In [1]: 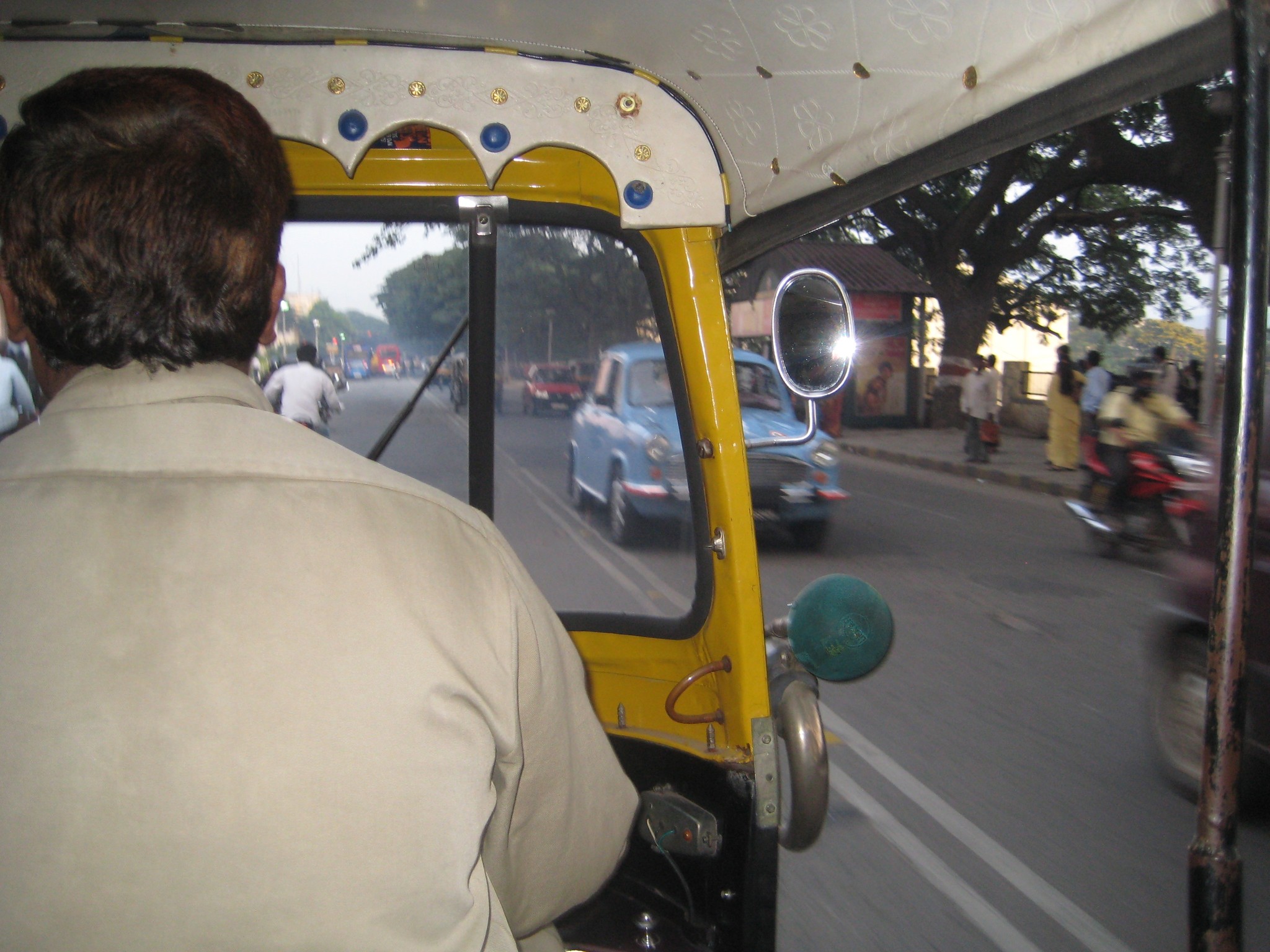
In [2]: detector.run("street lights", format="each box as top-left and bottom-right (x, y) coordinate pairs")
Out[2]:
(280, 301), (288, 365)
(313, 318), (322, 355)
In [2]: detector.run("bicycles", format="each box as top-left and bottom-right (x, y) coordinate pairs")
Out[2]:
(299, 404), (345, 441)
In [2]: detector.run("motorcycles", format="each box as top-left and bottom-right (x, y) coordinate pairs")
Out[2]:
(1063, 419), (1217, 564)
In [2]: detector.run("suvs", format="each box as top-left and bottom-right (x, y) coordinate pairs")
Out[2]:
(571, 339), (847, 553)
(521, 362), (582, 416)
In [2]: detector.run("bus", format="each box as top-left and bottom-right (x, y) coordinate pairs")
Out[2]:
(377, 342), (402, 377)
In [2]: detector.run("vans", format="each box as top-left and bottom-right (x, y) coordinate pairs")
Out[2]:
(451, 351), (502, 415)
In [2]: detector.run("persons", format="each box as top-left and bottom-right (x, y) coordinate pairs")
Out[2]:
(1098, 370), (1203, 536)
(1148, 345), (1180, 403)
(1077, 348), (1112, 435)
(957, 352), (998, 464)
(1055, 345), (1087, 376)
(986, 351), (1001, 424)
(1127, 355), (1211, 422)
(261, 342), (345, 437)
(1044, 362), (1087, 473)
(0, 65), (638, 951)
(0, 337), (40, 440)
(878, 360), (907, 417)
(859, 374), (884, 418)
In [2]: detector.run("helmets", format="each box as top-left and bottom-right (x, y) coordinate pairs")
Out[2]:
(1129, 358), (1164, 394)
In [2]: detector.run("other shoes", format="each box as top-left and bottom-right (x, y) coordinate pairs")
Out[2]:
(967, 448), (997, 464)
(1044, 460), (1063, 471)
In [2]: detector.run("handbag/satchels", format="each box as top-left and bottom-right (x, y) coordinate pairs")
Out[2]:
(980, 422), (999, 445)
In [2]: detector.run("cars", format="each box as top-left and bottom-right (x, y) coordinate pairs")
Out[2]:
(405, 355), (451, 386)
(345, 358), (371, 379)
(1140, 501), (1270, 818)
(323, 364), (347, 389)
